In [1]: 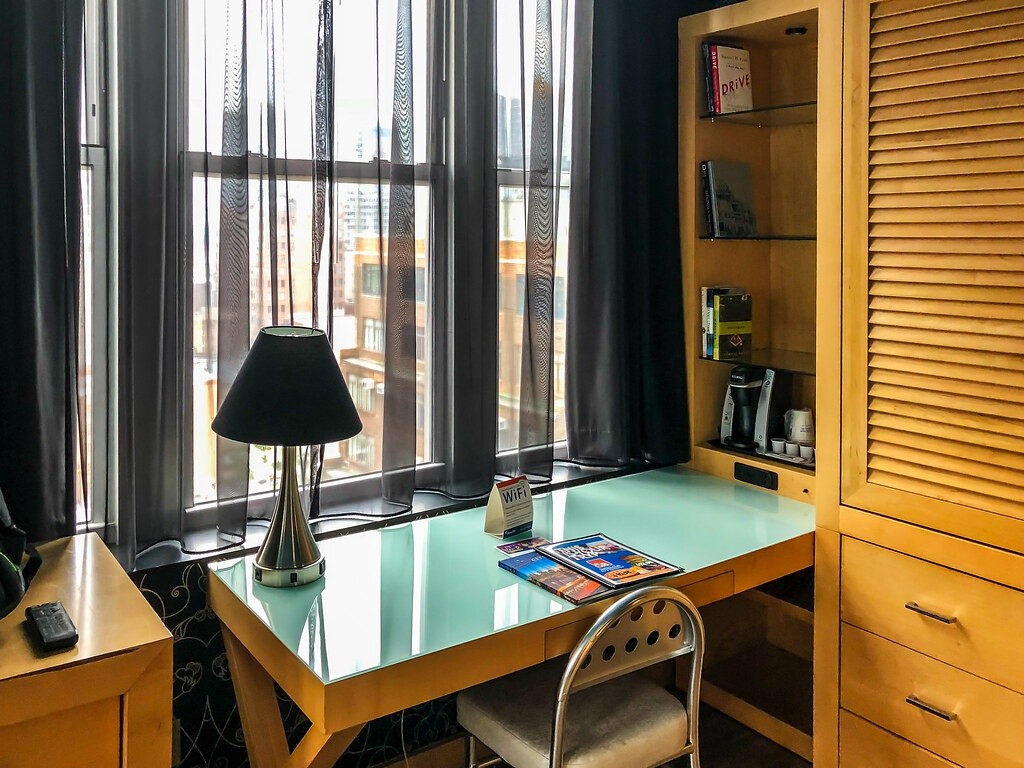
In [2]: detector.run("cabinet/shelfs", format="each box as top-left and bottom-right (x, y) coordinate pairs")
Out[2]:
(679, 0), (1024, 768)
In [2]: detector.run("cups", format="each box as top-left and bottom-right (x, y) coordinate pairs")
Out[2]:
(799, 445), (814, 462)
(784, 441), (799, 457)
(771, 438), (786, 453)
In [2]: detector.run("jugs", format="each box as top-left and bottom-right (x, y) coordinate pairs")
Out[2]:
(788, 409), (814, 446)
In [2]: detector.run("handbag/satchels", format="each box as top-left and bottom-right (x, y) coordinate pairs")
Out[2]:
(0, 492), (42, 619)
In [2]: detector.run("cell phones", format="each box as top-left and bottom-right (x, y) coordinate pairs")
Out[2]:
(24, 600), (79, 650)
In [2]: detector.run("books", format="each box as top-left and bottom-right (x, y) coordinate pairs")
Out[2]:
(701, 160), (758, 238)
(498, 533), (685, 606)
(700, 286), (752, 360)
(701, 40), (754, 120)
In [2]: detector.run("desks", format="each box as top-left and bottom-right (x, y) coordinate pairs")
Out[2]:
(207, 468), (817, 768)
(0, 533), (175, 768)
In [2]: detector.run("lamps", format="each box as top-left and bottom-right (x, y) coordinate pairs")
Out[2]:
(211, 326), (362, 589)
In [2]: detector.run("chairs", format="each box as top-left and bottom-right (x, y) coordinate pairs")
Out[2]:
(458, 586), (706, 768)
